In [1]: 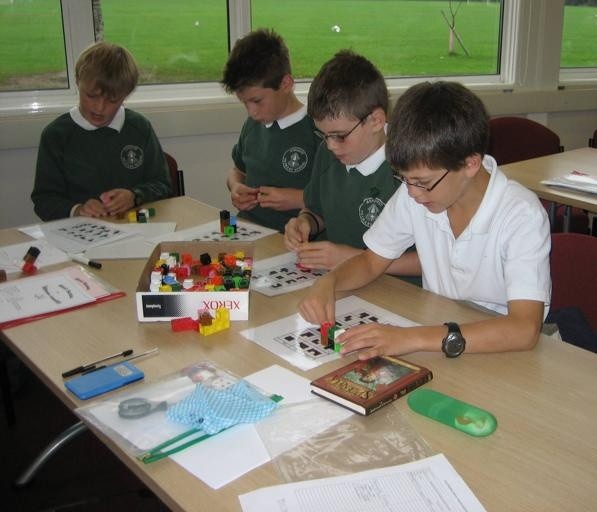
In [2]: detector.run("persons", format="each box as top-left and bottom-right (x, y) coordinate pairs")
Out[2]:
(283, 48), (422, 276)
(219, 27), (326, 233)
(32, 38), (173, 222)
(296, 81), (553, 364)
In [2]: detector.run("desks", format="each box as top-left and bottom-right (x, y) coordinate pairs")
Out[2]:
(496, 146), (597, 235)
(0, 193), (597, 512)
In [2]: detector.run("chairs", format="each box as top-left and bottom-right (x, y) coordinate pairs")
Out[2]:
(156, 151), (185, 198)
(486, 116), (588, 230)
(541, 229), (597, 355)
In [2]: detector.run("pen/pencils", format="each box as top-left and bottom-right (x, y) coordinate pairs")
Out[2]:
(72, 255), (101, 269)
(62, 348), (160, 378)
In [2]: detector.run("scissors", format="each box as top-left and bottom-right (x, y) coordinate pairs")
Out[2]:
(119, 398), (167, 419)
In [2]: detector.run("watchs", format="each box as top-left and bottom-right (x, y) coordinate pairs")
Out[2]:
(441, 321), (465, 359)
(131, 188), (144, 207)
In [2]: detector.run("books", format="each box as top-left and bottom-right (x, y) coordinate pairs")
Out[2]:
(309, 352), (433, 418)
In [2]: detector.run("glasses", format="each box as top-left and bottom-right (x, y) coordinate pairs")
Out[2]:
(313, 111), (372, 143)
(392, 167), (450, 195)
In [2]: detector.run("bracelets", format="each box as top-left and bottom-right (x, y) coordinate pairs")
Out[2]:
(298, 211), (321, 235)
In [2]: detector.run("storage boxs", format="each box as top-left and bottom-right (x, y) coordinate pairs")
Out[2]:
(134, 238), (254, 323)
